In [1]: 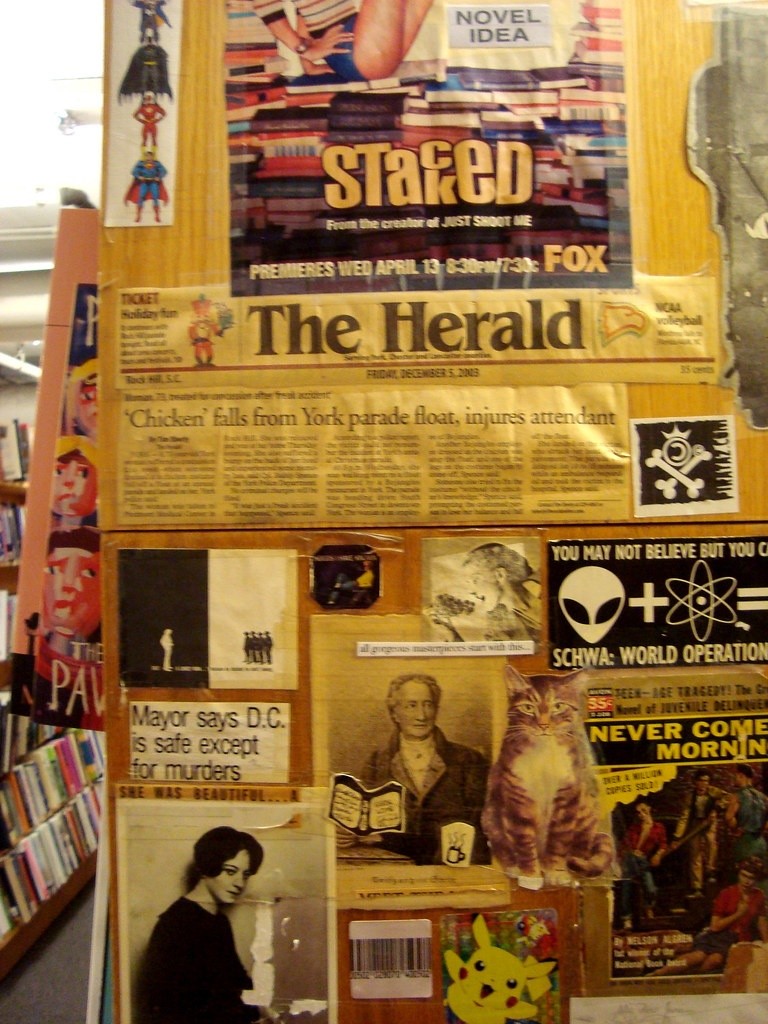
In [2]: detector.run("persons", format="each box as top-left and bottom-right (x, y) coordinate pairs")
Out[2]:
(669, 768), (731, 900)
(356, 673), (491, 867)
(428, 543), (542, 643)
(617, 800), (667, 933)
(724, 766), (766, 880)
(137, 828), (283, 1024)
(645, 853), (767, 978)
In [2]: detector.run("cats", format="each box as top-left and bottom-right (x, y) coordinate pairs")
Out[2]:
(480, 664), (617, 889)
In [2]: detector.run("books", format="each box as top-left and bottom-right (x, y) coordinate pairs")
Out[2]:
(226, 1), (633, 278)
(0, 416), (105, 937)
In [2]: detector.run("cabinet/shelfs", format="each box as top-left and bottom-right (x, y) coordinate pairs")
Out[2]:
(1, 351), (107, 991)
